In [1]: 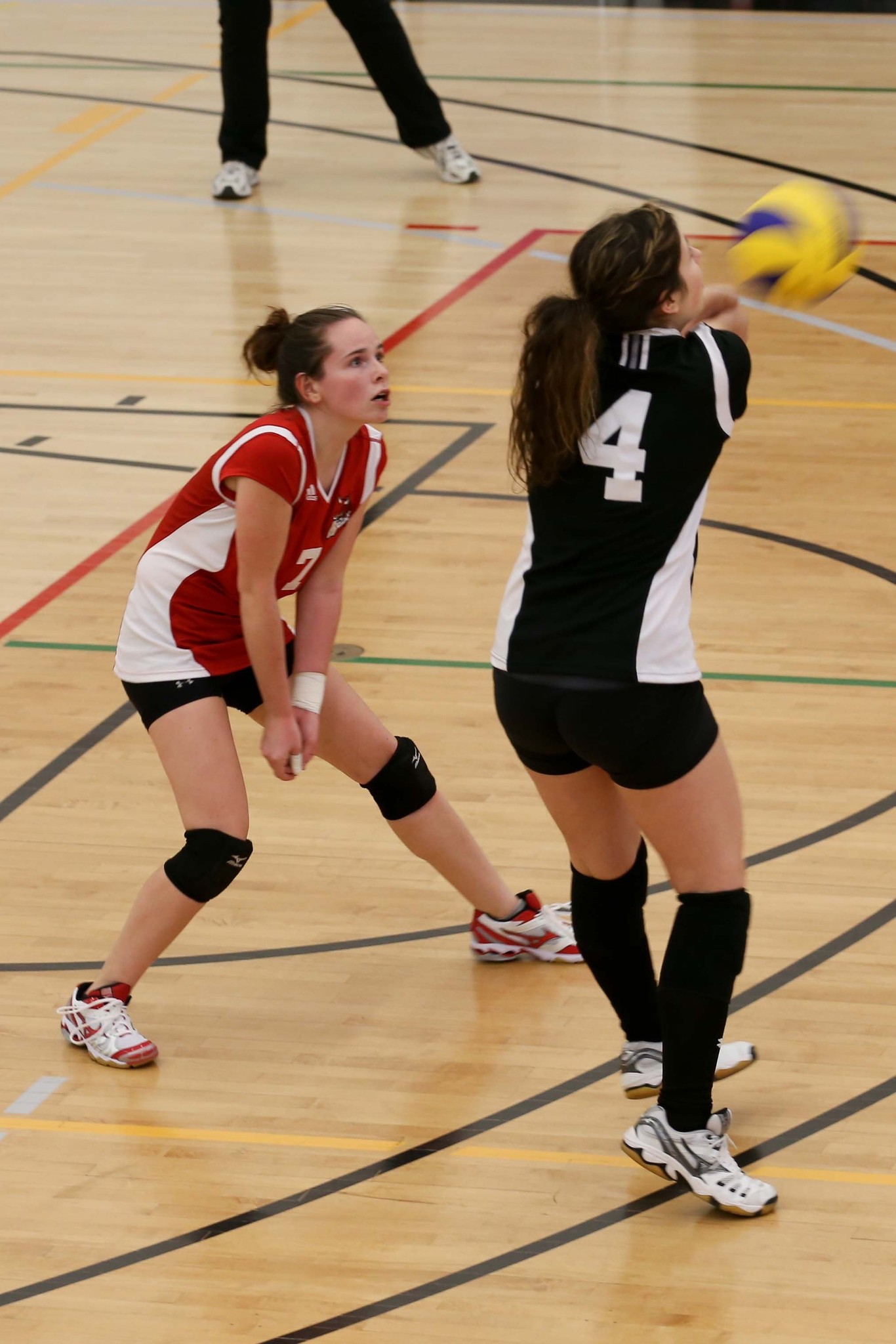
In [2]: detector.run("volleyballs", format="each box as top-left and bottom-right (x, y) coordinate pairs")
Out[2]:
(728, 180), (865, 308)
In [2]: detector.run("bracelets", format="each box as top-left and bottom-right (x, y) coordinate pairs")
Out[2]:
(289, 672), (326, 715)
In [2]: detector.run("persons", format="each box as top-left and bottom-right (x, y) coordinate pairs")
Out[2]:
(55, 303), (584, 1069)
(212, 0), (480, 199)
(489, 200), (780, 1216)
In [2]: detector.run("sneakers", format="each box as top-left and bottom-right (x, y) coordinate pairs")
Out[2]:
(619, 1107), (778, 1216)
(212, 160), (259, 198)
(413, 133), (482, 184)
(620, 1039), (757, 1098)
(57, 981), (158, 1068)
(471, 888), (586, 962)
